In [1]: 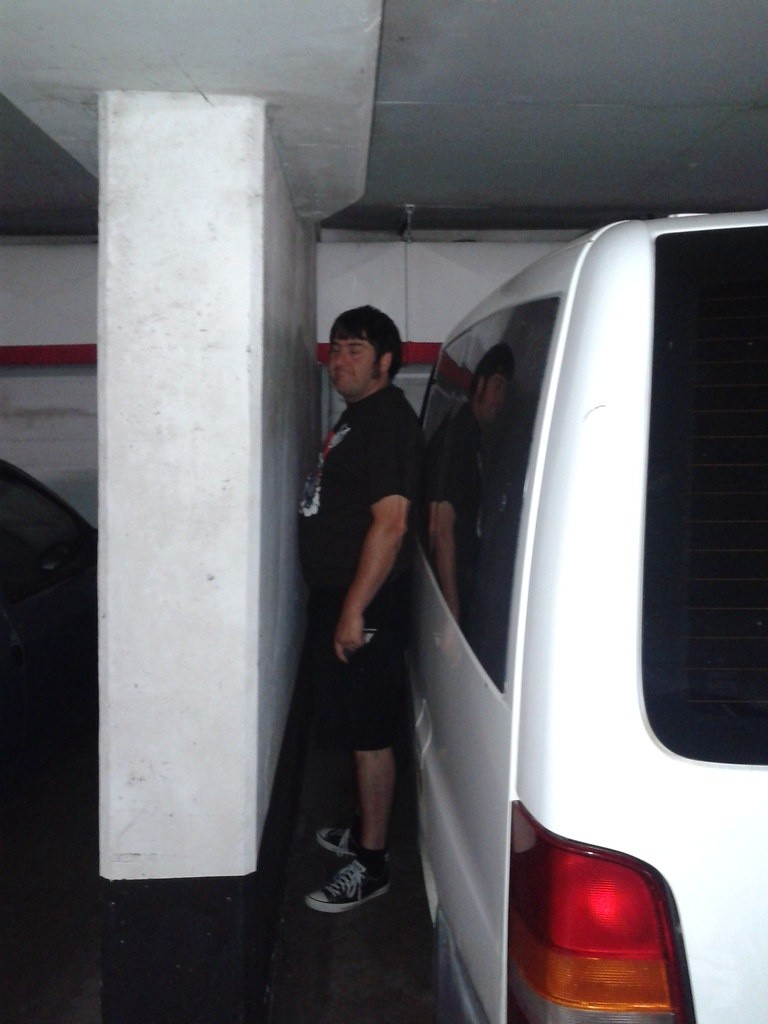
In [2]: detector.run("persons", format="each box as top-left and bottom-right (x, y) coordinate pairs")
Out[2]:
(299, 304), (422, 911)
(423, 341), (514, 626)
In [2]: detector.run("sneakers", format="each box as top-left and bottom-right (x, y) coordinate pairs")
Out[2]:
(304, 860), (391, 913)
(316, 827), (390, 863)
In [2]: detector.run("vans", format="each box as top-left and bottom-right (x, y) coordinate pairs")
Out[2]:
(391, 210), (768, 1024)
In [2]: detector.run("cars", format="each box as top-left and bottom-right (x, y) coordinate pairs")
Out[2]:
(0, 458), (101, 911)
